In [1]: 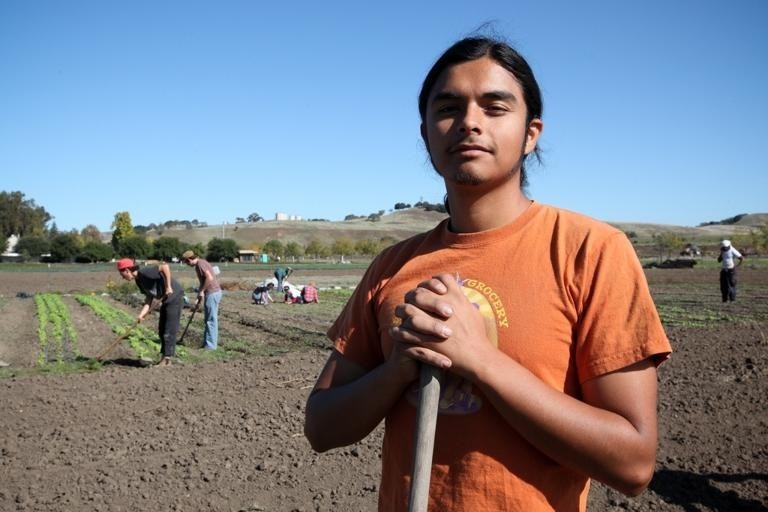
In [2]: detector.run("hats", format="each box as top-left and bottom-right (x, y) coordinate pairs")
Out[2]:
(181, 250), (194, 263)
(117, 257), (133, 270)
(722, 240), (729, 247)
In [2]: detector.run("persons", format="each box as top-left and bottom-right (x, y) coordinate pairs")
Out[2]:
(302, 20), (675, 512)
(300, 280), (320, 304)
(274, 266), (293, 292)
(181, 250), (223, 351)
(282, 285), (302, 304)
(717, 239), (745, 303)
(252, 282), (277, 306)
(117, 258), (185, 367)
